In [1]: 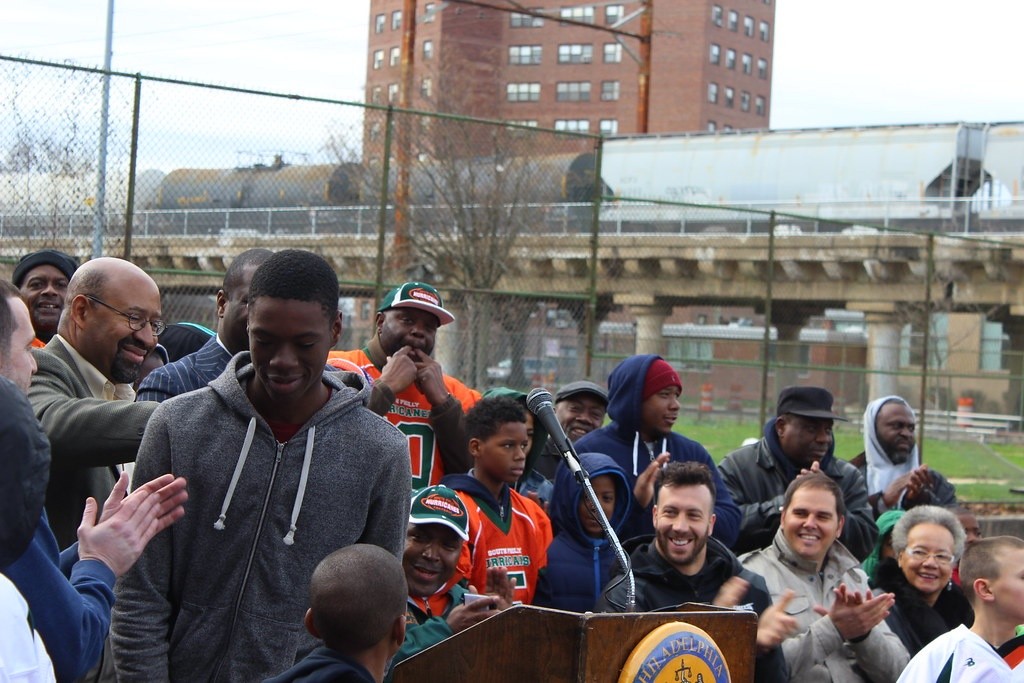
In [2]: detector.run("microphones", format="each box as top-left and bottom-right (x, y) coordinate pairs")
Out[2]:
(527, 388), (584, 485)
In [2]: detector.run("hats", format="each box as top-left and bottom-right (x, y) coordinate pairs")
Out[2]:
(11, 249), (80, 292)
(777, 386), (848, 422)
(555, 380), (609, 410)
(408, 482), (471, 543)
(377, 281), (454, 325)
(642, 357), (683, 404)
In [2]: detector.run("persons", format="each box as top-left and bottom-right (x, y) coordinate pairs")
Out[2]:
(12, 248), (274, 683)
(718, 385), (1024, 683)
(263, 544), (409, 683)
(0, 375), (56, 683)
(444, 354), (798, 683)
(109, 250), (412, 683)
(0, 278), (188, 683)
(383, 484), (517, 683)
(326, 281), (478, 492)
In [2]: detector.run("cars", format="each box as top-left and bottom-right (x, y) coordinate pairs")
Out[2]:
(486, 354), (560, 389)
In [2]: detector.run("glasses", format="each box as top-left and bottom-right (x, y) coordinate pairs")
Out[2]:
(83, 295), (168, 336)
(903, 544), (956, 565)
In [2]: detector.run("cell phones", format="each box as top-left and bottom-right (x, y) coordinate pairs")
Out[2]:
(463, 593), (490, 611)
